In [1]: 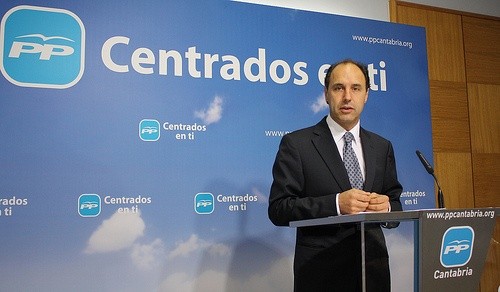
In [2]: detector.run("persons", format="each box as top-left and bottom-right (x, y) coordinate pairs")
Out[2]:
(267, 58), (403, 292)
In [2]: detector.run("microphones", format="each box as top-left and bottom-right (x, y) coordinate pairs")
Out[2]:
(415, 150), (444, 209)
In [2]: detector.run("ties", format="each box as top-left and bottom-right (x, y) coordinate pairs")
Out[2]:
(342, 131), (363, 190)
